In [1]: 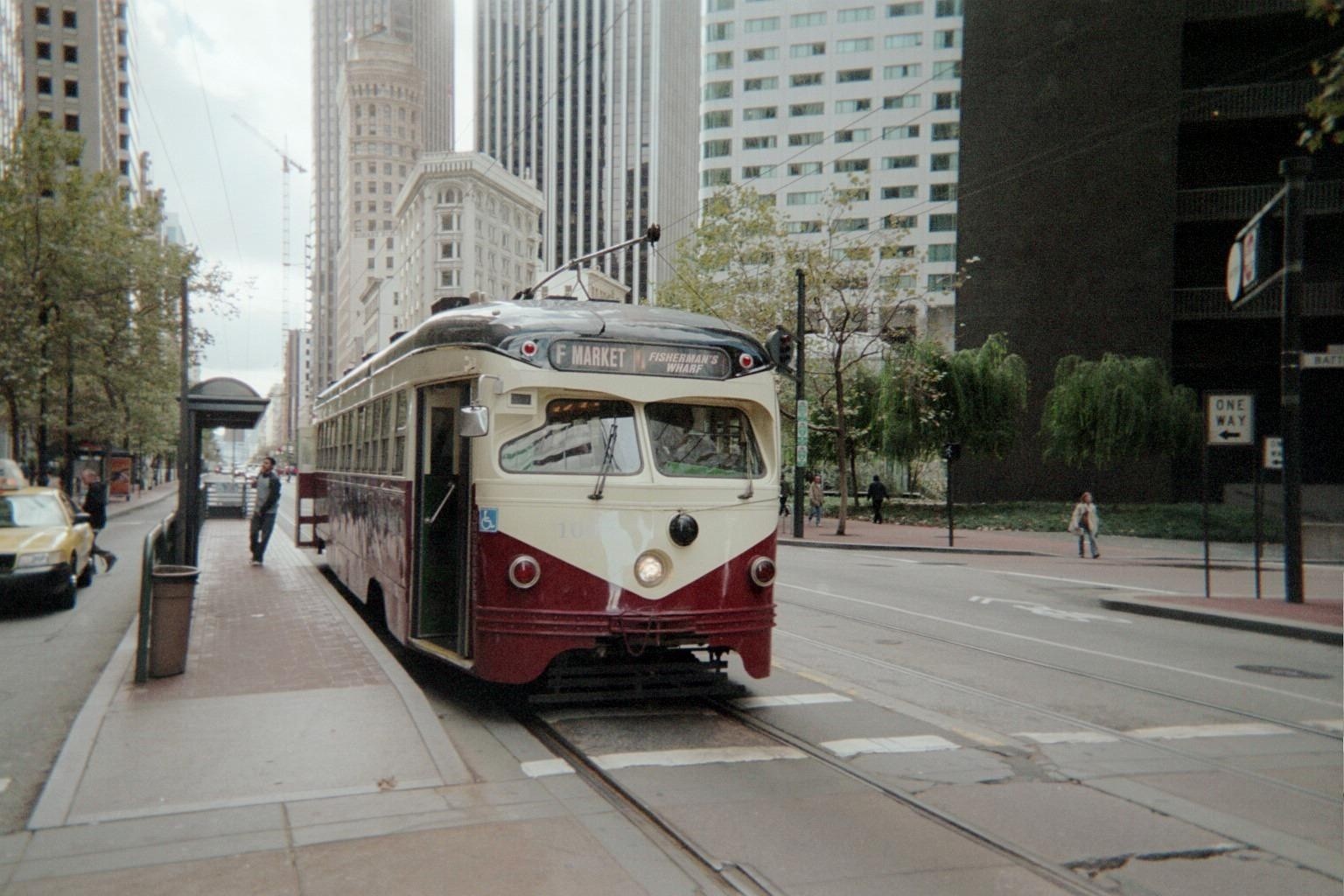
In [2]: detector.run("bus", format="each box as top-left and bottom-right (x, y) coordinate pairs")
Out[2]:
(308, 291), (785, 713)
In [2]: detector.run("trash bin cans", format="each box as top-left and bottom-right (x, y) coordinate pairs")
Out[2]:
(148, 565), (200, 678)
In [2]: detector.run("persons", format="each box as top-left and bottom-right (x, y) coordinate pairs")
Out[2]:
(808, 474), (824, 528)
(867, 474), (890, 523)
(249, 455), (283, 564)
(653, 405), (723, 468)
(1067, 491), (1101, 560)
(79, 467), (121, 574)
(779, 472), (792, 516)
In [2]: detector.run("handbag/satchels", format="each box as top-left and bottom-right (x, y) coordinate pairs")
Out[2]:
(1071, 526), (1084, 536)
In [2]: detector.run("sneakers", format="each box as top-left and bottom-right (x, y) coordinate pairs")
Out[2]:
(1092, 553), (1100, 559)
(104, 552), (117, 573)
(1078, 556), (1085, 558)
(250, 558), (264, 567)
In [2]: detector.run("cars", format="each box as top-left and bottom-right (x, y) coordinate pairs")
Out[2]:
(2, 458), (93, 613)
